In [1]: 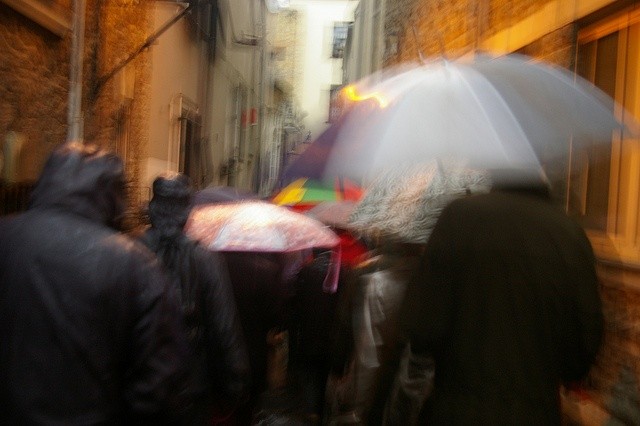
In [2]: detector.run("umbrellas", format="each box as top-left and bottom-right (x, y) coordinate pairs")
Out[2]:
(278, 176), (364, 208)
(341, 162), (504, 244)
(323, 53), (635, 190)
(191, 188), (261, 204)
(187, 199), (340, 252)
(276, 113), (344, 185)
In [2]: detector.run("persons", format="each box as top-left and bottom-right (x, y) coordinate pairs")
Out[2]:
(137, 176), (252, 424)
(291, 230), (354, 423)
(388, 173), (604, 424)
(340, 237), (424, 425)
(1, 141), (185, 426)
(225, 252), (290, 425)
